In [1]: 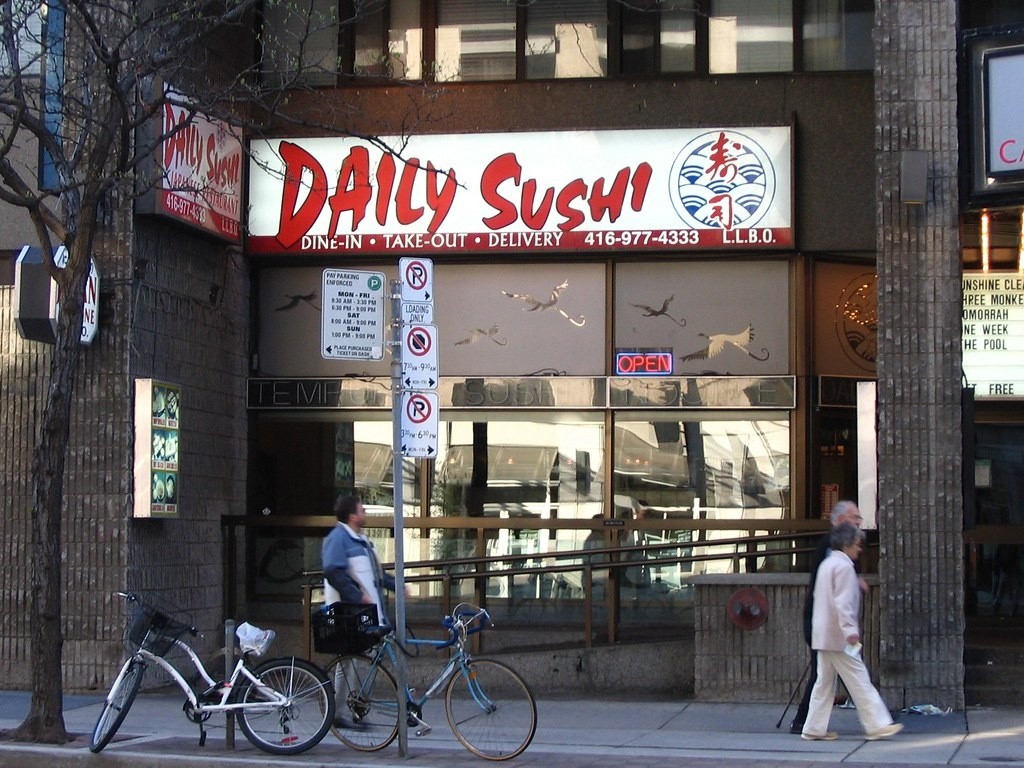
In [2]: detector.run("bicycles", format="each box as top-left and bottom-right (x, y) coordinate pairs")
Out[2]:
(89, 590), (337, 756)
(317, 600), (538, 761)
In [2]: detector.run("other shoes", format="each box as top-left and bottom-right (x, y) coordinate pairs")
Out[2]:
(865, 723), (904, 741)
(802, 731), (838, 740)
(790, 721), (803, 733)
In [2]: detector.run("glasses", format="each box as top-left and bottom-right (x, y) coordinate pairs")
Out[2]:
(853, 541), (864, 549)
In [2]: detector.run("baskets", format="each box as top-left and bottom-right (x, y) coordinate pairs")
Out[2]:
(129, 595), (194, 657)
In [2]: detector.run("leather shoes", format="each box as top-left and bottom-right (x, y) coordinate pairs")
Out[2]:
(333, 716), (369, 730)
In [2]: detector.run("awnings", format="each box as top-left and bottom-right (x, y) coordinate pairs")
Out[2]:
(354, 442), (395, 489)
(439, 444), (560, 488)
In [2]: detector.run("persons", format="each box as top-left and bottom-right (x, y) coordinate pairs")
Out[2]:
(582, 516), (608, 597)
(322, 497), (413, 729)
(791, 503), (903, 741)
